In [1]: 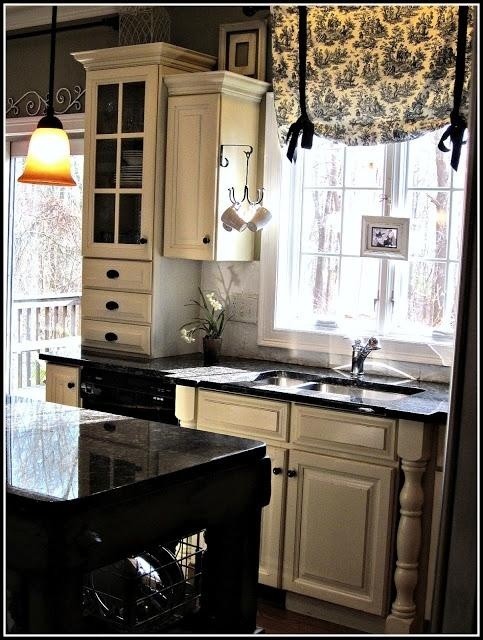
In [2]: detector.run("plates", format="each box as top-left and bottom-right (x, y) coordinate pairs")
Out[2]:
(87, 545), (188, 628)
(111, 148), (143, 188)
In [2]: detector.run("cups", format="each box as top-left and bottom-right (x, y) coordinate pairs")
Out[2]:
(220, 206), (247, 233)
(247, 207), (272, 233)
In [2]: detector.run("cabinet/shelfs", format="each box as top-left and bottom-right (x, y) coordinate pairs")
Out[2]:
(162, 69), (267, 262)
(69, 45), (200, 357)
(39, 360), (443, 635)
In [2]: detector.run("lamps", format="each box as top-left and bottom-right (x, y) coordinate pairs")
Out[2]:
(16, 1), (74, 187)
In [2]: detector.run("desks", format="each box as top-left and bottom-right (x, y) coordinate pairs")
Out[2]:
(6, 394), (266, 636)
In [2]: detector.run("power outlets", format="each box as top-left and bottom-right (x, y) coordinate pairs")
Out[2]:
(228, 295), (257, 324)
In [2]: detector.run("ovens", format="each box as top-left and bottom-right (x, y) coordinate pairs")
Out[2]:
(79, 369), (177, 427)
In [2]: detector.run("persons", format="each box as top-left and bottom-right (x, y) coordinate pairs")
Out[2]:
(387, 230), (395, 245)
(375, 231), (392, 247)
(381, 233), (387, 240)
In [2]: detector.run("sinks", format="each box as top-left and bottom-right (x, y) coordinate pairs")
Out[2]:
(249, 369), (314, 388)
(298, 377), (426, 401)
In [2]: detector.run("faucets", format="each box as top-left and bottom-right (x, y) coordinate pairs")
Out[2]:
(350, 336), (381, 375)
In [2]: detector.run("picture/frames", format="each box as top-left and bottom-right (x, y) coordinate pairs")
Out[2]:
(216, 19), (265, 83)
(360, 213), (409, 260)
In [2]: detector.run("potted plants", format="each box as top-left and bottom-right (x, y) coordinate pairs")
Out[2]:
(181, 287), (233, 359)
